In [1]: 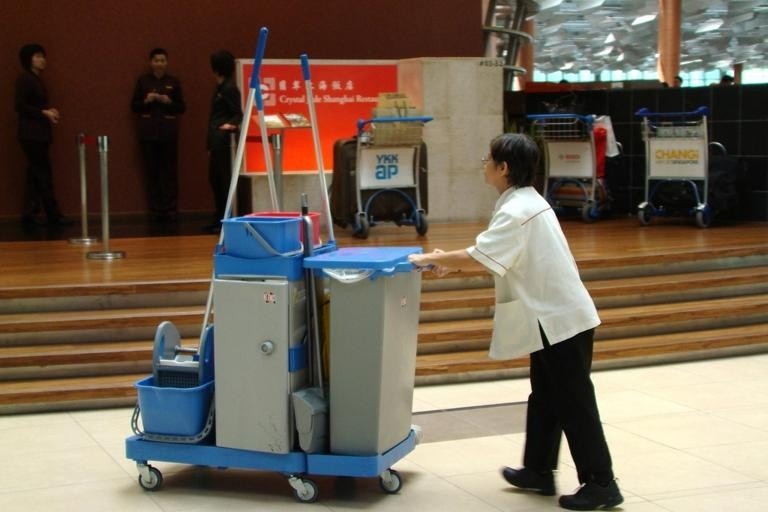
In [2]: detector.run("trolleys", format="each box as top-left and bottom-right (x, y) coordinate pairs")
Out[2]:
(528, 111), (612, 220)
(99, 247), (436, 495)
(345, 113), (431, 234)
(630, 103), (738, 227)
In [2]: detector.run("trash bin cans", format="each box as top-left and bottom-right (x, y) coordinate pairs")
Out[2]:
(303, 246), (423, 457)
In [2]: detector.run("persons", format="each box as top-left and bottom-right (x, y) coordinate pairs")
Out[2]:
(13, 42), (70, 231)
(411, 133), (626, 510)
(131, 46), (181, 221)
(204, 47), (251, 230)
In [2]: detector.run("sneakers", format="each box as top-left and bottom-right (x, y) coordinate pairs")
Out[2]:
(146, 215), (183, 234)
(201, 219), (222, 233)
(21, 211), (78, 233)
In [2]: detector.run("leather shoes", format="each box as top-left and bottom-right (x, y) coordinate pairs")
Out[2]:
(503, 466), (556, 495)
(559, 480), (624, 510)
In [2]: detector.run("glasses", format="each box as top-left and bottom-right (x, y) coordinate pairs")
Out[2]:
(481, 156), (496, 163)
